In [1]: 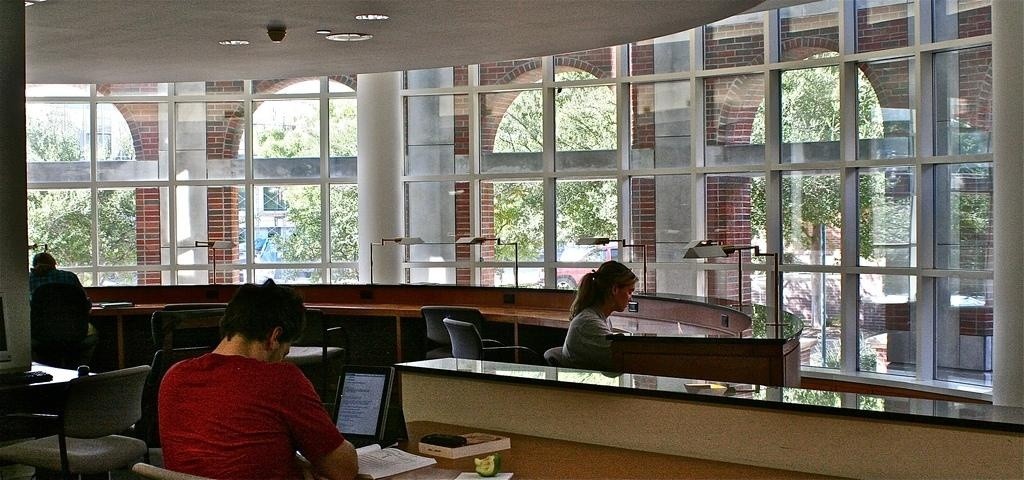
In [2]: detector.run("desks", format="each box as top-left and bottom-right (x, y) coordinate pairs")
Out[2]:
(295, 419), (854, 480)
(81, 300), (740, 408)
(0, 361), (97, 393)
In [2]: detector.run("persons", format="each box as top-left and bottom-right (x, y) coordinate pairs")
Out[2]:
(157, 283), (358, 479)
(29, 252), (90, 363)
(562, 260), (640, 364)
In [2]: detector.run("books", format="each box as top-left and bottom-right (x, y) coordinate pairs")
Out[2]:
(419, 432), (511, 459)
(354, 443), (437, 480)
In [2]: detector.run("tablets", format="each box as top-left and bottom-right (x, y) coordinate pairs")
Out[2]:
(332, 365), (395, 442)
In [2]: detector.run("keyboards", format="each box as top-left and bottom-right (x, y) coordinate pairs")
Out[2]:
(1, 371), (54, 386)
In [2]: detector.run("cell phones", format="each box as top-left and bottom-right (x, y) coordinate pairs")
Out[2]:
(420, 433), (466, 447)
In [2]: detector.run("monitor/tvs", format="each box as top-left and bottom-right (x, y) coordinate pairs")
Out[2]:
(0, 293), (16, 363)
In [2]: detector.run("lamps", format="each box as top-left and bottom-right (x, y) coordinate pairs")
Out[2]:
(680, 239), (779, 308)
(370, 237), (424, 285)
(576, 238), (647, 294)
(455, 237), (518, 288)
(195, 241), (237, 265)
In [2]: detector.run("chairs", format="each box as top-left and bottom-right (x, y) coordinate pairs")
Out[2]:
(132, 462), (217, 480)
(0, 364), (151, 480)
(281, 308), (345, 402)
(443, 318), (541, 365)
(141, 303), (230, 422)
(421, 305), (507, 361)
